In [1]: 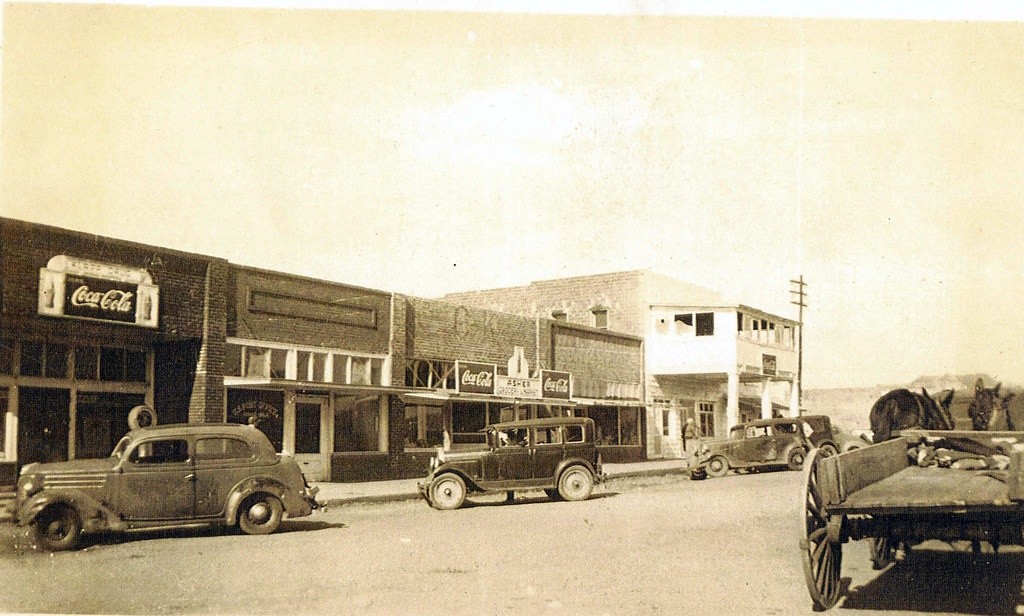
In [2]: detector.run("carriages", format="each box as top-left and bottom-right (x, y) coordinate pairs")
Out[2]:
(798, 378), (1023, 610)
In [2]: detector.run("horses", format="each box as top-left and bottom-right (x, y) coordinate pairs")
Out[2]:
(869, 378), (1016, 445)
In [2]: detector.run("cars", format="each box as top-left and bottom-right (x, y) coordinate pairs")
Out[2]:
(11, 421), (323, 550)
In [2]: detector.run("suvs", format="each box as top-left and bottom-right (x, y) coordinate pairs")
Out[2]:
(416, 416), (609, 510)
(794, 416), (841, 464)
(695, 413), (819, 478)
